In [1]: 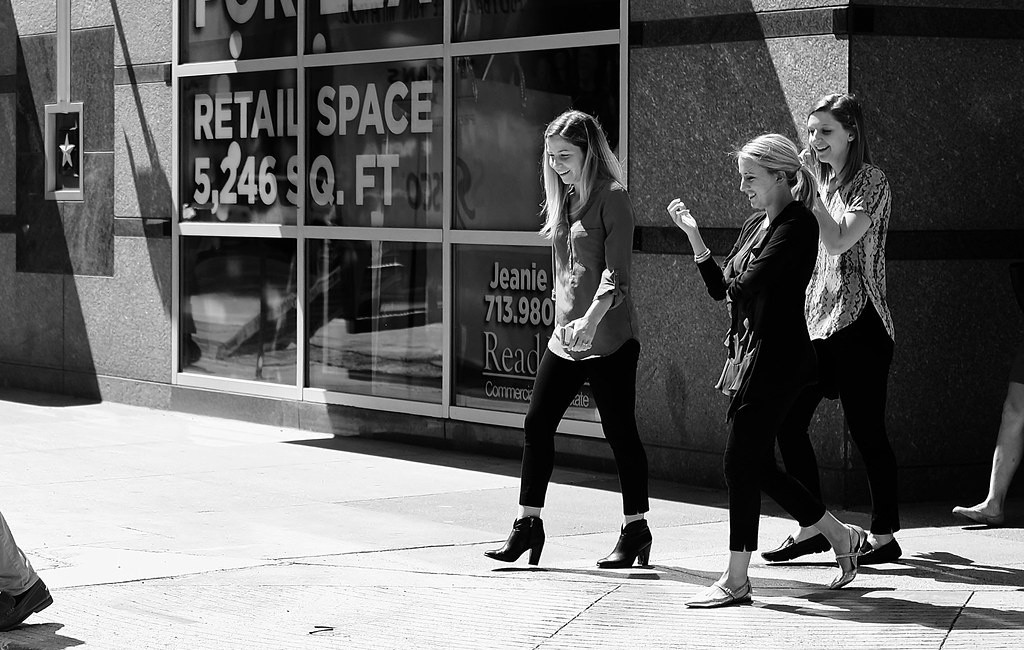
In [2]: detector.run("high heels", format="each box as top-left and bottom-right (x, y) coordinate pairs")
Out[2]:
(484, 516), (545, 565)
(597, 519), (652, 568)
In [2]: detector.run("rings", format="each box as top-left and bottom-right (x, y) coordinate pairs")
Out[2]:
(582, 342), (592, 348)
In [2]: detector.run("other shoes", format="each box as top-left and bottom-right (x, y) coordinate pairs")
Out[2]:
(857, 538), (902, 564)
(953, 506), (1005, 526)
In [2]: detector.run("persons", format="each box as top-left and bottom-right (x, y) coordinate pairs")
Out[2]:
(952, 157), (1024, 527)
(0, 0), (101, 632)
(485, 111), (652, 569)
(761, 95), (902, 567)
(667, 133), (868, 609)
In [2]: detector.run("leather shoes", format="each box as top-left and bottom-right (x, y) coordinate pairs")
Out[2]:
(684, 576), (753, 607)
(830, 523), (868, 589)
(0, 578), (53, 632)
(761, 533), (832, 562)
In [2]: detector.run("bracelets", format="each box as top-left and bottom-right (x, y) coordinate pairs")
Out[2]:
(695, 249), (711, 261)
(816, 191), (820, 197)
